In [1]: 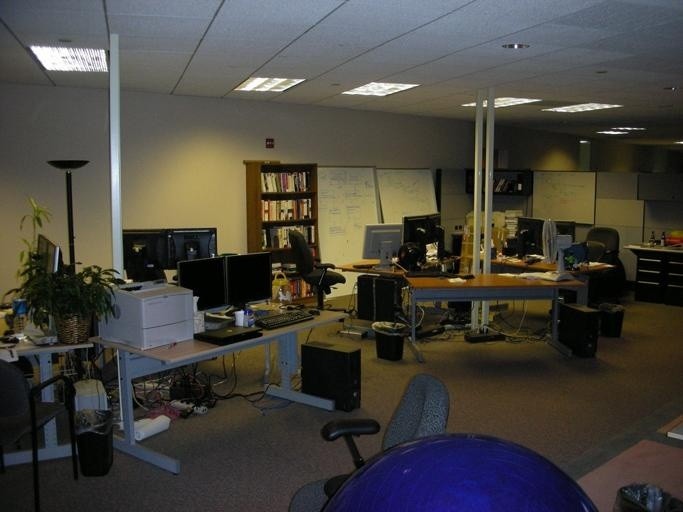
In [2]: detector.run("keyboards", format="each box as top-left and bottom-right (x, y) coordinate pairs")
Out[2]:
(255, 311), (314, 329)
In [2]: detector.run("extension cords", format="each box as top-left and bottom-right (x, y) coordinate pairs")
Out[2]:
(171, 400), (208, 416)
(463, 331), (507, 344)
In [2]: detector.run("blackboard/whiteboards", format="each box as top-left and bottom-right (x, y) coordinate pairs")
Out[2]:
(531, 169), (596, 225)
(377, 169), (437, 223)
(318, 166), (382, 300)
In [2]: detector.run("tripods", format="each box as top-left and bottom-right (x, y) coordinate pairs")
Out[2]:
(177, 256), (226, 319)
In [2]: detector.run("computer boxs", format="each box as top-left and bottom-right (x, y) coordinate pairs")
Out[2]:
(560, 302), (600, 358)
(358, 275), (400, 322)
(301, 341), (361, 410)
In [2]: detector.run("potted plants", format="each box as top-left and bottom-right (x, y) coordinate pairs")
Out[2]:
(20, 266), (126, 345)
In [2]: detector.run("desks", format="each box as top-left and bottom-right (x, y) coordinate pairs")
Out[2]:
(335, 255), (462, 328)
(487, 247), (615, 330)
(405, 274), (584, 363)
(87, 304), (350, 476)
(0, 330), (95, 467)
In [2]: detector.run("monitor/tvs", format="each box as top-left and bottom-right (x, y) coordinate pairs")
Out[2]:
(403, 213), (446, 261)
(167, 228), (217, 281)
(122, 228), (166, 282)
(363, 223), (403, 271)
(513, 217), (575, 263)
(226, 251), (273, 318)
(36, 235), (65, 333)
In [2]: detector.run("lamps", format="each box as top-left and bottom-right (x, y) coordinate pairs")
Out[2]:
(48, 160), (90, 274)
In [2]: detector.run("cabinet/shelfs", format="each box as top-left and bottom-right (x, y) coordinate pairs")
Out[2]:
(625, 249), (683, 307)
(464, 167), (533, 196)
(246, 158), (320, 305)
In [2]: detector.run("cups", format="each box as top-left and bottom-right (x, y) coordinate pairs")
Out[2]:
(12, 298), (26, 314)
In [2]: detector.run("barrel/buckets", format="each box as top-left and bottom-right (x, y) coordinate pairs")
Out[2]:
(272, 271), (289, 300)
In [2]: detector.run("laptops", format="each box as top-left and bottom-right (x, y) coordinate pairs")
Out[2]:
(194, 327), (263, 347)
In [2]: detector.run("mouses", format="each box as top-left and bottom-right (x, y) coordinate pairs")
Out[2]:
(309, 310), (320, 316)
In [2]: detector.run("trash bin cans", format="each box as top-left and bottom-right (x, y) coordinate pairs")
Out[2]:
(75, 410), (114, 477)
(600, 303), (624, 338)
(373, 321), (406, 361)
(616, 485), (683, 512)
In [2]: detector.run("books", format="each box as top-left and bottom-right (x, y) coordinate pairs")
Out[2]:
(260, 171), (315, 300)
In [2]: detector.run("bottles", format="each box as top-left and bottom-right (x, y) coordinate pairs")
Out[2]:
(243, 309), (255, 328)
(649, 231), (655, 247)
(661, 232), (666, 246)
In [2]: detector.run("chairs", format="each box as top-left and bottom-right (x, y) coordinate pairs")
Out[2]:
(287, 373), (451, 507)
(586, 226), (620, 258)
(319, 434), (599, 511)
(286, 229), (346, 314)
(1, 358), (79, 511)
